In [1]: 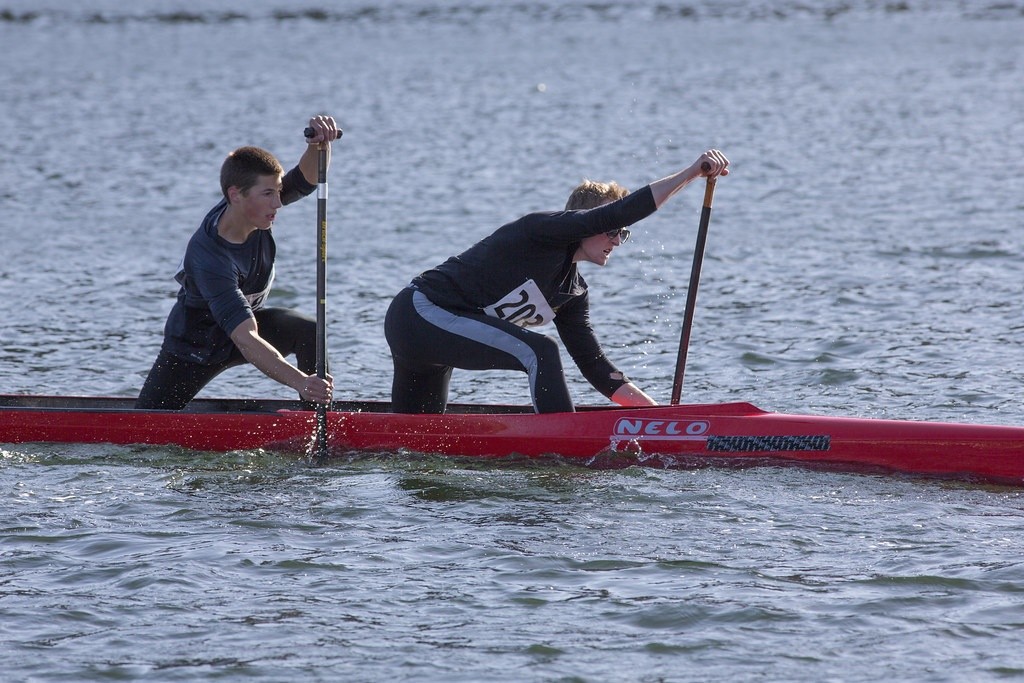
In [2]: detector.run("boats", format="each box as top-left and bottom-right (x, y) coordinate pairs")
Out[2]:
(0, 386), (1024, 481)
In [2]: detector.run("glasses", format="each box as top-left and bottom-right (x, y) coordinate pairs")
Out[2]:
(605, 229), (631, 244)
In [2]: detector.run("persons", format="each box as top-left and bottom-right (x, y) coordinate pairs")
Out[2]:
(134, 114), (338, 411)
(385, 148), (730, 416)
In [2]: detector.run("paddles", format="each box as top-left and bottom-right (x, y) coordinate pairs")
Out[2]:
(302, 124), (348, 468)
(667, 162), (721, 408)
